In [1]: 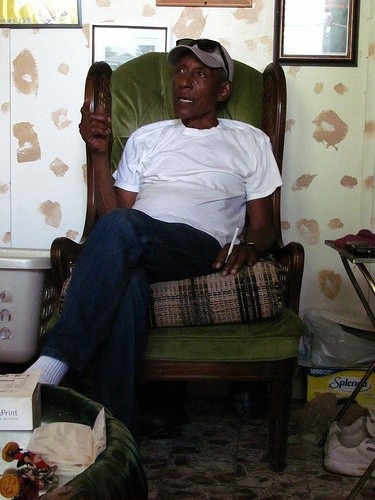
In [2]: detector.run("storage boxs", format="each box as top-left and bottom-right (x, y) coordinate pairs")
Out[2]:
(1, 372), (42, 430)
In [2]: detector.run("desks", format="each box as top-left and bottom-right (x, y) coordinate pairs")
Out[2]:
(0, 374), (148, 500)
(318, 240), (375, 443)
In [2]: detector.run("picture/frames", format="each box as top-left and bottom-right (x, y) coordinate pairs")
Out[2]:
(275, 0), (361, 68)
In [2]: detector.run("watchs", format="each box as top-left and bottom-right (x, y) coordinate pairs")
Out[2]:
(238, 241), (259, 255)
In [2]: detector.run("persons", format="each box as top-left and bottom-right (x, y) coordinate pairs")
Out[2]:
(20, 37), (284, 445)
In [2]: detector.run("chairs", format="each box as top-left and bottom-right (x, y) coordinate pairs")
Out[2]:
(48, 51), (304, 474)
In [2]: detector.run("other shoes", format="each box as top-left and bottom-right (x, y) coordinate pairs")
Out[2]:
(323, 408), (375, 478)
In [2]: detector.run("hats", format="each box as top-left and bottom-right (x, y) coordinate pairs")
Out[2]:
(168, 40), (235, 82)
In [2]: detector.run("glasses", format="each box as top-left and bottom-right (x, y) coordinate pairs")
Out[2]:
(175, 38), (230, 81)
